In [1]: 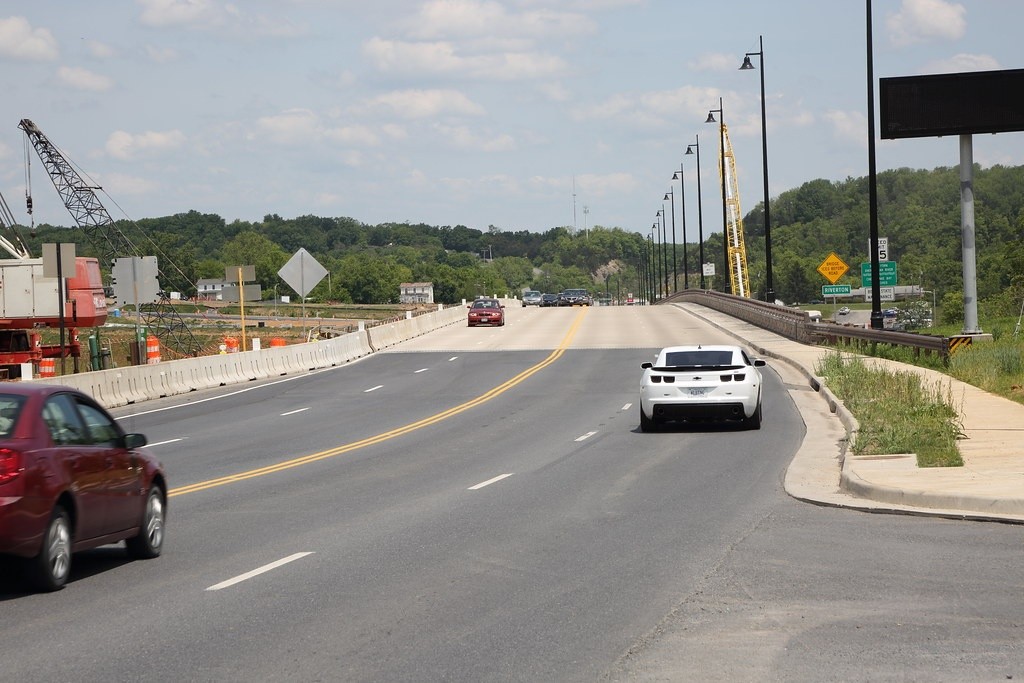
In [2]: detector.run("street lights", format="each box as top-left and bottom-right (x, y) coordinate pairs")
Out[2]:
(655, 204), (668, 298)
(737, 35), (775, 305)
(684, 134), (706, 288)
(663, 186), (677, 293)
(670, 163), (689, 289)
(646, 233), (657, 302)
(704, 97), (733, 294)
(652, 218), (662, 300)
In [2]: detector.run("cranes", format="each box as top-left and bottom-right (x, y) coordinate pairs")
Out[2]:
(16, 116), (222, 362)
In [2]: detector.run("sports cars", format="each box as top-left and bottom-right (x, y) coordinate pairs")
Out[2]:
(639, 345), (766, 429)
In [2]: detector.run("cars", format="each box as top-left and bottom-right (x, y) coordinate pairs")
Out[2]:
(522, 291), (544, 308)
(467, 298), (506, 327)
(840, 307), (850, 315)
(882, 307), (911, 318)
(0, 382), (170, 590)
(541, 294), (558, 307)
(558, 288), (593, 307)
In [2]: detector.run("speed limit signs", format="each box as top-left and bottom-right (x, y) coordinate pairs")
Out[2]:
(868, 238), (890, 263)
